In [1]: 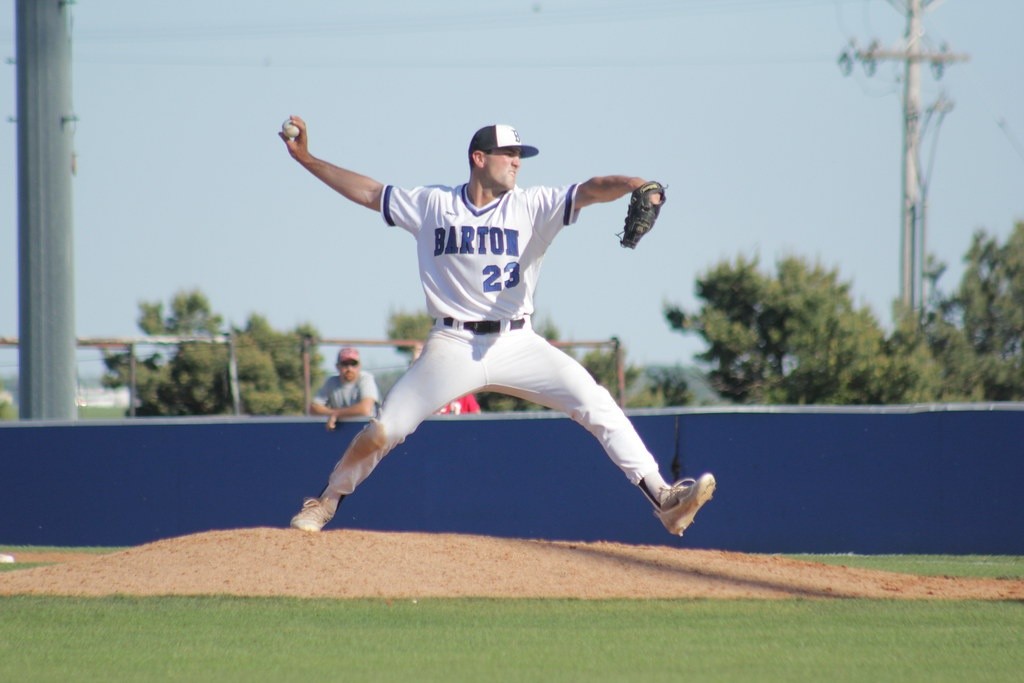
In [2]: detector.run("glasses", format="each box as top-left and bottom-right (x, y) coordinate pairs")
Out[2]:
(342, 361), (358, 366)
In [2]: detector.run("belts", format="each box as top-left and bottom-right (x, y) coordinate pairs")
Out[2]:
(433, 317), (526, 335)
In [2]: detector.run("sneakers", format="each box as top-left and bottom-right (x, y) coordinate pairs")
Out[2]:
(653, 472), (717, 538)
(290, 497), (335, 532)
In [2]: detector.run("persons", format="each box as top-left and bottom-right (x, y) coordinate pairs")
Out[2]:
(278, 114), (717, 537)
(310, 348), (382, 433)
(435, 394), (482, 416)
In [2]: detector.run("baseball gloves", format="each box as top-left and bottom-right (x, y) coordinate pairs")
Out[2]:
(620, 181), (667, 250)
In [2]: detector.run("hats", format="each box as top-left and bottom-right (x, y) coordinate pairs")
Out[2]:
(338, 347), (360, 363)
(468, 125), (540, 165)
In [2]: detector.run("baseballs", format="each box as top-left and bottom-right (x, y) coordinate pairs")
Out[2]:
(283, 119), (300, 138)
